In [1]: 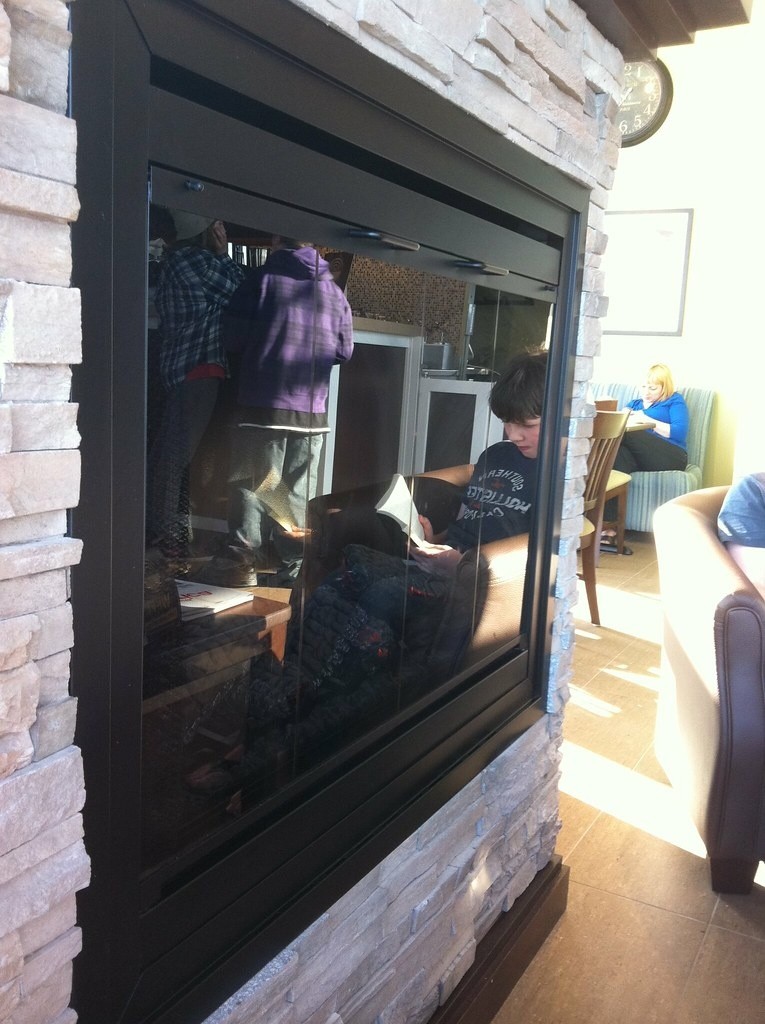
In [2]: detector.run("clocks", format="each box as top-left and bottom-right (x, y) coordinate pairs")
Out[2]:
(615, 56), (672, 147)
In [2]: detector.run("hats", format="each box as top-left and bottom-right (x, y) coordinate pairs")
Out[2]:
(169, 208), (215, 240)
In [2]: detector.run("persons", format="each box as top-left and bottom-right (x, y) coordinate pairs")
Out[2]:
(151, 213), (353, 587)
(600, 364), (690, 546)
(715, 469), (765, 603)
(185, 347), (551, 796)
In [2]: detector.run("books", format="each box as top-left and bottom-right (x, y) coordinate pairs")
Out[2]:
(376, 473), (424, 554)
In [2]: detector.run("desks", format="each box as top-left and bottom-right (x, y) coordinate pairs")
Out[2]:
(625, 422), (655, 433)
(143, 579), (291, 715)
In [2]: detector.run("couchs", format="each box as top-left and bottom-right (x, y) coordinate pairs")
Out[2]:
(287, 463), (530, 689)
(654, 485), (765, 889)
(587, 383), (716, 532)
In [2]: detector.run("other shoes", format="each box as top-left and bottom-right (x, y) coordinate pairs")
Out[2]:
(600, 531), (617, 547)
(263, 569), (296, 590)
(189, 556), (259, 589)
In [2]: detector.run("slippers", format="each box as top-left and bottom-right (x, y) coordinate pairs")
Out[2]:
(181, 756), (251, 798)
(223, 782), (264, 818)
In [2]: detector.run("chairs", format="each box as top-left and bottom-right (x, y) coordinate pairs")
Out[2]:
(579, 408), (632, 627)
(594, 399), (631, 554)
